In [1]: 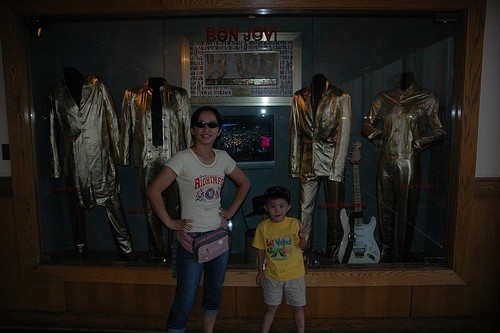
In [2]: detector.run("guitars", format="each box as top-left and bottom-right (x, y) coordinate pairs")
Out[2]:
(337, 141), (381, 264)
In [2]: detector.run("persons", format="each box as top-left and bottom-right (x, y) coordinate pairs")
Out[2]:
(147, 107), (252, 333)
(119, 77), (195, 268)
(288, 73), (353, 267)
(45, 67), (143, 263)
(252, 186), (312, 333)
(361, 72), (453, 262)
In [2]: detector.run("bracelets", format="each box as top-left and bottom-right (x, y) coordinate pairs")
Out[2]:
(258, 269), (262, 273)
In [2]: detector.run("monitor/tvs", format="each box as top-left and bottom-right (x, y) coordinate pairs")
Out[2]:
(211, 115), (274, 162)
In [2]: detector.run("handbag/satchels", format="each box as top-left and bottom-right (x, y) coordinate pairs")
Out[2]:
(177, 219), (230, 263)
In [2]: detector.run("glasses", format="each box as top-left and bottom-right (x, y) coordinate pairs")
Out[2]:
(194, 120), (219, 128)
(265, 186), (290, 201)
